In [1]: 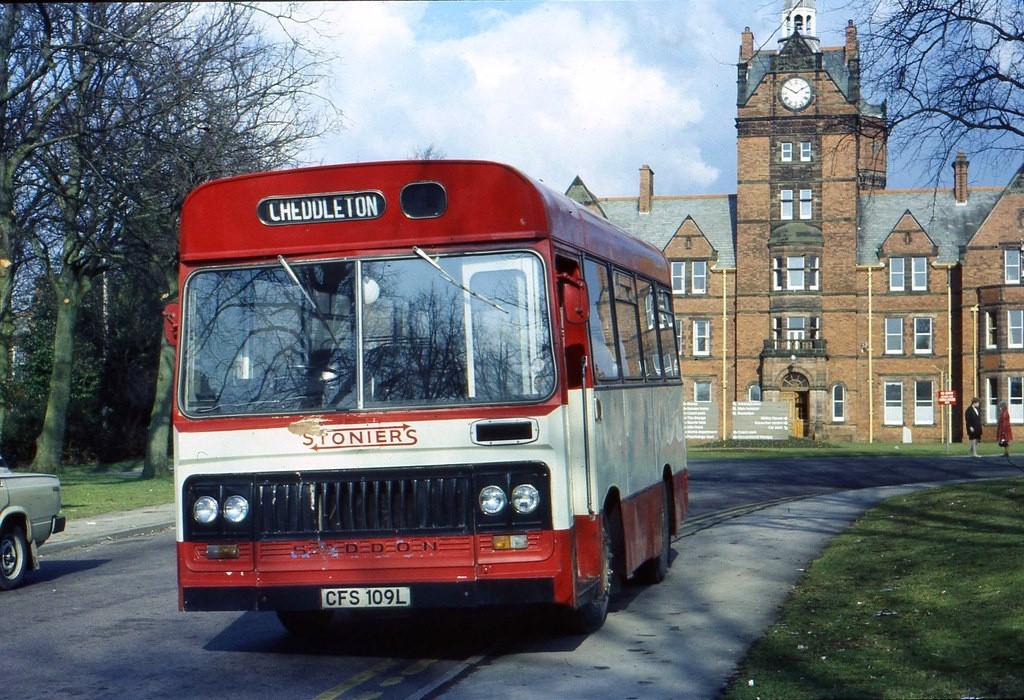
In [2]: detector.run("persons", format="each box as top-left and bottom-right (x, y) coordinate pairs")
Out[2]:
(965, 398), (982, 457)
(996, 401), (1013, 458)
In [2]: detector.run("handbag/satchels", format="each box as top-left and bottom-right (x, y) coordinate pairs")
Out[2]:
(999, 439), (1009, 447)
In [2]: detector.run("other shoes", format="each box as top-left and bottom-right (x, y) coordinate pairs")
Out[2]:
(973, 454), (981, 458)
(1001, 453), (1009, 457)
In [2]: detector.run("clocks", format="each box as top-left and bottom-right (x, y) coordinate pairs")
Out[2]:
(778, 76), (814, 112)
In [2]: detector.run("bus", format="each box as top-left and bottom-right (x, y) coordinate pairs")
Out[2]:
(160, 158), (690, 645)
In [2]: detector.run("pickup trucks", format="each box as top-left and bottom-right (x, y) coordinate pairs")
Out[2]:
(0, 472), (66, 591)
